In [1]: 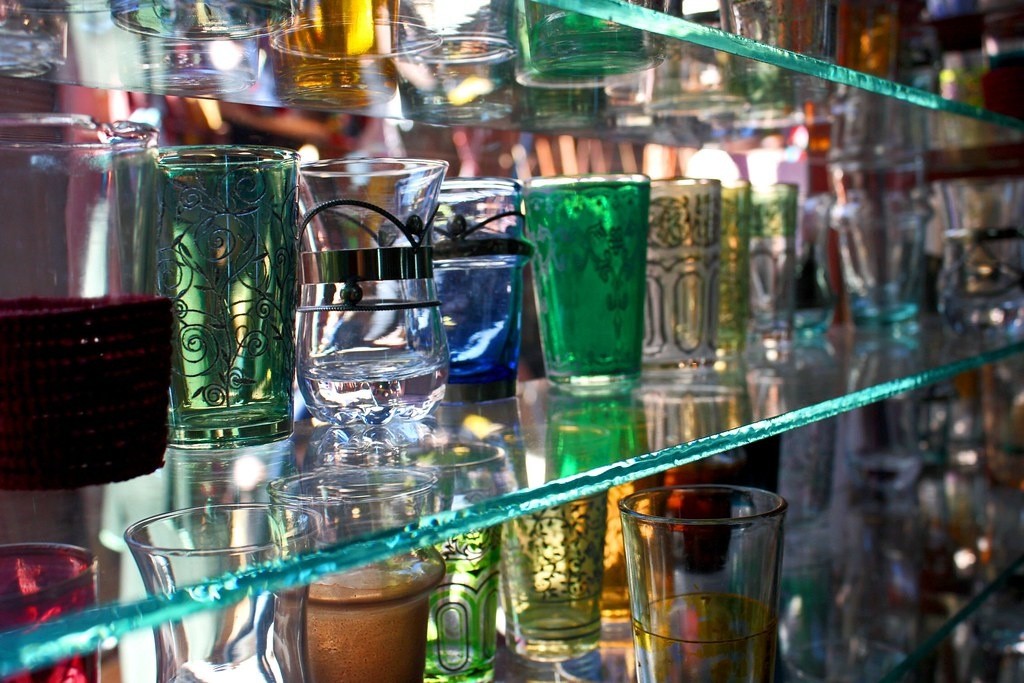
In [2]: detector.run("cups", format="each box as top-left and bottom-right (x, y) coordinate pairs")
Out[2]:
(0, 0), (828, 122)
(0, 123), (1023, 485)
(0, 470), (785, 683)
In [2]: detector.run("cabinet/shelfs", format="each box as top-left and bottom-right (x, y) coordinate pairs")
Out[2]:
(0, 0), (1024, 683)
